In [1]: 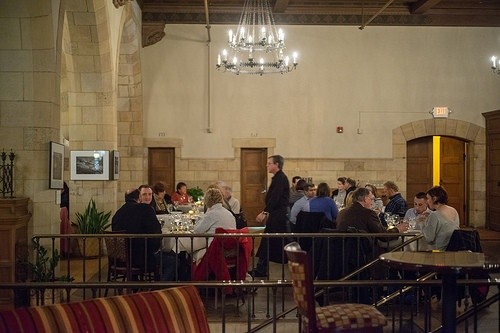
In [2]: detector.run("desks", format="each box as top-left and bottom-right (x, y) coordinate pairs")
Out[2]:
(380, 251), (500, 333)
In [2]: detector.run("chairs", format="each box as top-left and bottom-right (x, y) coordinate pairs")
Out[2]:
(191, 228), (253, 309)
(444, 230), (482, 252)
(297, 211), (335, 250)
(284, 241), (388, 333)
(103, 231), (159, 297)
(317, 228), (359, 304)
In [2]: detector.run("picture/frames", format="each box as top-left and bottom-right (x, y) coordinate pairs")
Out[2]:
(70, 150), (110, 181)
(113, 150), (120, 180)
(49, 141), (65, 189)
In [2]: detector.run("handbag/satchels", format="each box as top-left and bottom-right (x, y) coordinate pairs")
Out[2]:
(233, 213), (244, 230)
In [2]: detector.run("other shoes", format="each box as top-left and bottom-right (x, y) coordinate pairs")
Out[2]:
(248, 269), (267, 276)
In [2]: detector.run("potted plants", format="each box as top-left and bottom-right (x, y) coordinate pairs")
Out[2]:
(71, 197), (111, 256)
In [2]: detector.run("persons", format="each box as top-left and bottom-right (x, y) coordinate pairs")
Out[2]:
(289, 176), (460, 300)
(111, 182), (240, 282)
(247, 155), (291, 278)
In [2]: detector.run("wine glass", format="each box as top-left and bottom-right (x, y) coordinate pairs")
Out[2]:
(384, 212), (417, 231)
(158, 196), (203, 233)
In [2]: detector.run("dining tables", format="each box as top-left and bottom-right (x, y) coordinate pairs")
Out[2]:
(157, 200), (204, 281)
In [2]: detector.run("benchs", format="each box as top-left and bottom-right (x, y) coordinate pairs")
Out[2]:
(0, 286), (210, 333)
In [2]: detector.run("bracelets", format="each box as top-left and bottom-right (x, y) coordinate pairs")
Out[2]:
(263, 212), (267, 215)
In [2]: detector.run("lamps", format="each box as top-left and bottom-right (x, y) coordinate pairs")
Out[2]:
(429, 107), (452, 119)
(216, 0), (298, 75)
(491, 55), (500, 75)
